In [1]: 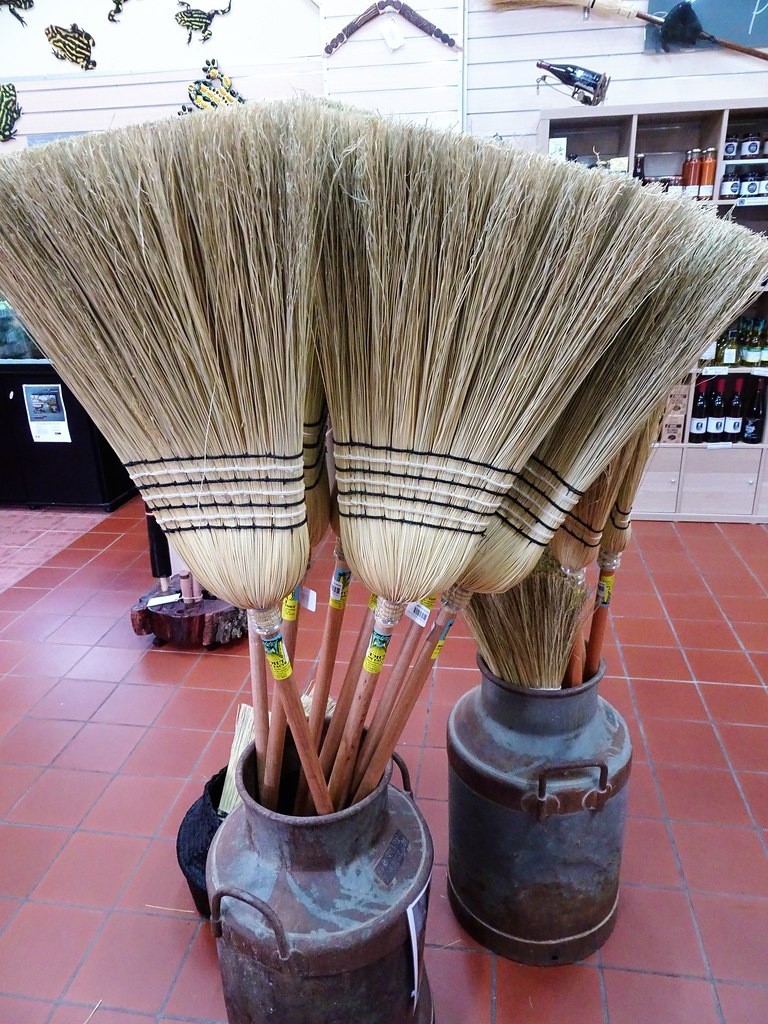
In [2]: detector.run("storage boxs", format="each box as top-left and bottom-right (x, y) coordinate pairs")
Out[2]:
(661, 416), (685, 443)
(548, 137), (567, 159)
(666, 385), (690, 415)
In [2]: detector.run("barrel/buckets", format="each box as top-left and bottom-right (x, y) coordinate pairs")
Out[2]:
(446, 638), (634, 968)
(205, 716), (435, 1024)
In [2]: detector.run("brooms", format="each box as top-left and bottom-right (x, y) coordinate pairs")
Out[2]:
(0, 96), (767, 813)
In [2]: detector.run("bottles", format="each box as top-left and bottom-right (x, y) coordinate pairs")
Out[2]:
(723, 132), (768, 160)
(718, 171), (768, 200)
(644, 176), (683, 191)
(536, 58), (606, 91)
(698, 315), (768, 368)
(689, 375), (766, 444)
(682, 148), (701, 185)
(634, 153), (644, 184)
(700, 147), (717, 185)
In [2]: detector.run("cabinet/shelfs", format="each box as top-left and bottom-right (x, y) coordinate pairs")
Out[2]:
(535, 97), (768, 526)
(0, 294), (141, 513)
